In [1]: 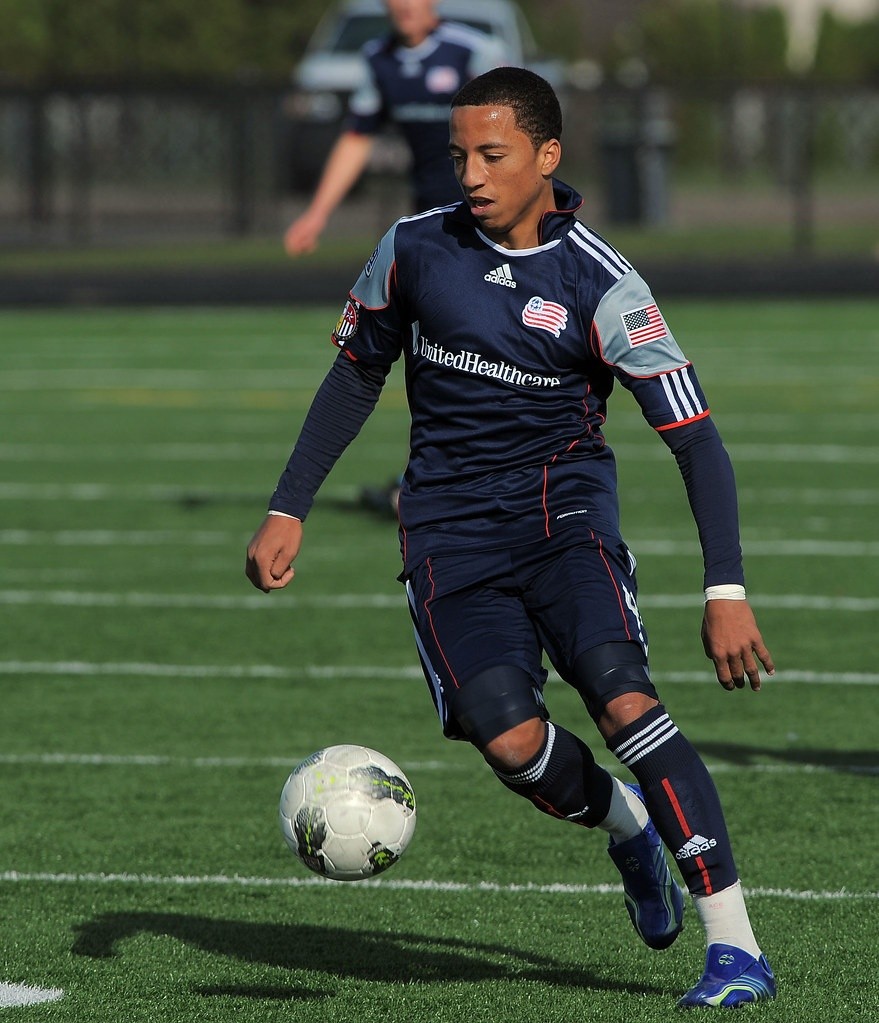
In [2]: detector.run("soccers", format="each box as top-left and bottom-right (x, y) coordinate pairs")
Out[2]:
(275, 744), (419, 883)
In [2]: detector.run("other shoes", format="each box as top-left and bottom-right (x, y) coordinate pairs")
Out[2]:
(363, 476), (398, 512)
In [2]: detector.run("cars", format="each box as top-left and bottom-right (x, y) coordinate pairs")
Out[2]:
(270, 0), (526, 197)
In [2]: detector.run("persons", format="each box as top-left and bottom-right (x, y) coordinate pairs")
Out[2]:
(283, 0), (500, 511)
(245, 66), (776, 1009)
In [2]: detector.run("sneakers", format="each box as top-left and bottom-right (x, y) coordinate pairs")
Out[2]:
(676, 943), (778, 1008)
(606, 783), (686, 949)
